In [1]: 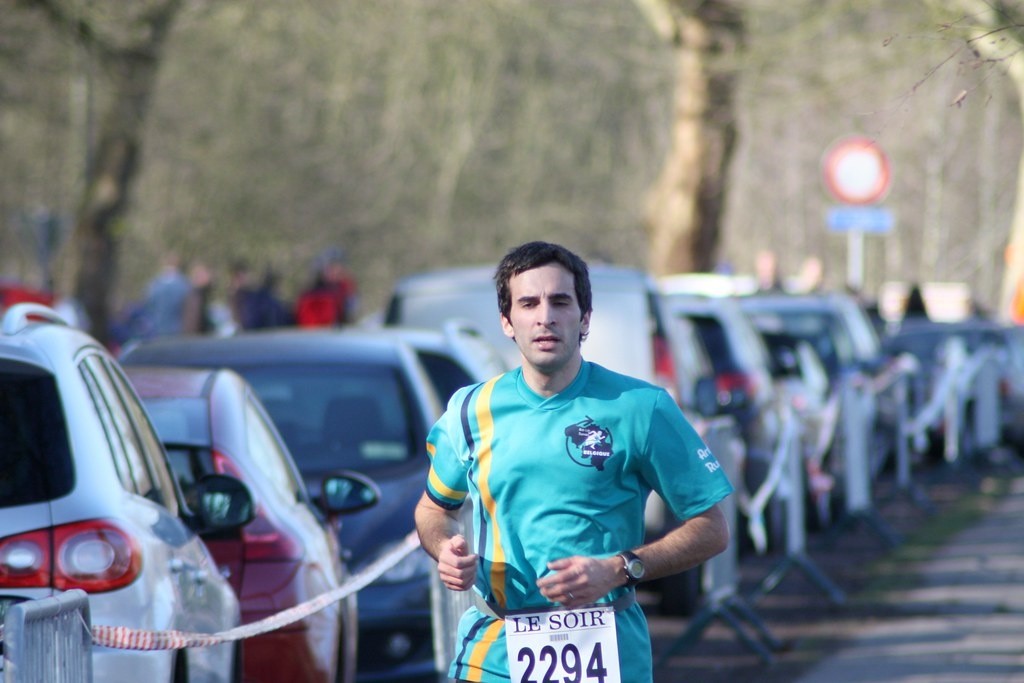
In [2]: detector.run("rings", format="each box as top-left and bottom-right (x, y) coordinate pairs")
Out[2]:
(569, 592), (574, 600)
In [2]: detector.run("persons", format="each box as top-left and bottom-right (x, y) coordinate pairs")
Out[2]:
(413, 240), (736, 683)
(113, 245), (357, 338)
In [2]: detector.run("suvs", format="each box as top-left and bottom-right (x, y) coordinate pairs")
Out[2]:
(382, 261), (750, 623)
(0, 304), (252, 683)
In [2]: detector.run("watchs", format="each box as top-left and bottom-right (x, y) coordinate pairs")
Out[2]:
(619, 551), (645, 585)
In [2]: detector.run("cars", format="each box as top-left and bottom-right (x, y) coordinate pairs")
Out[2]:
(107, 331), (463, 683)
(667, 290), (1024, 568)
(116, 367), (384, 683)
